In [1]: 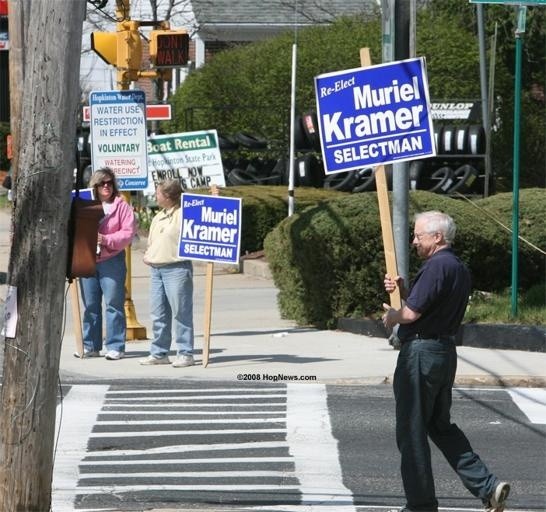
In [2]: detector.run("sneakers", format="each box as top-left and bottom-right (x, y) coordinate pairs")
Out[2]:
(143, 354), (168, 364)
(172, 354), (194, 366)
(390, 504), (413, 512)
(483, 482), (510, 512)
(74, 349), (98, 357)
(105, 350), (124, 360)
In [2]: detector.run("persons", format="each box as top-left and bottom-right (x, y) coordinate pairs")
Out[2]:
(143, 175), (197, 369)
(71, 165), (139, 362)
(379, 207), (512, 512)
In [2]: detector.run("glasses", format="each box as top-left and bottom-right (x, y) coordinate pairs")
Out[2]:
(98, 181), (112, 186)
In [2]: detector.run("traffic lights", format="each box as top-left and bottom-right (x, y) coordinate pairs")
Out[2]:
(90, 31), (118, 68)
(150, 29), (189, 70)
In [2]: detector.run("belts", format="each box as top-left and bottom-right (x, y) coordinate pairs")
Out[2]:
(405, 333), (455, 341)
